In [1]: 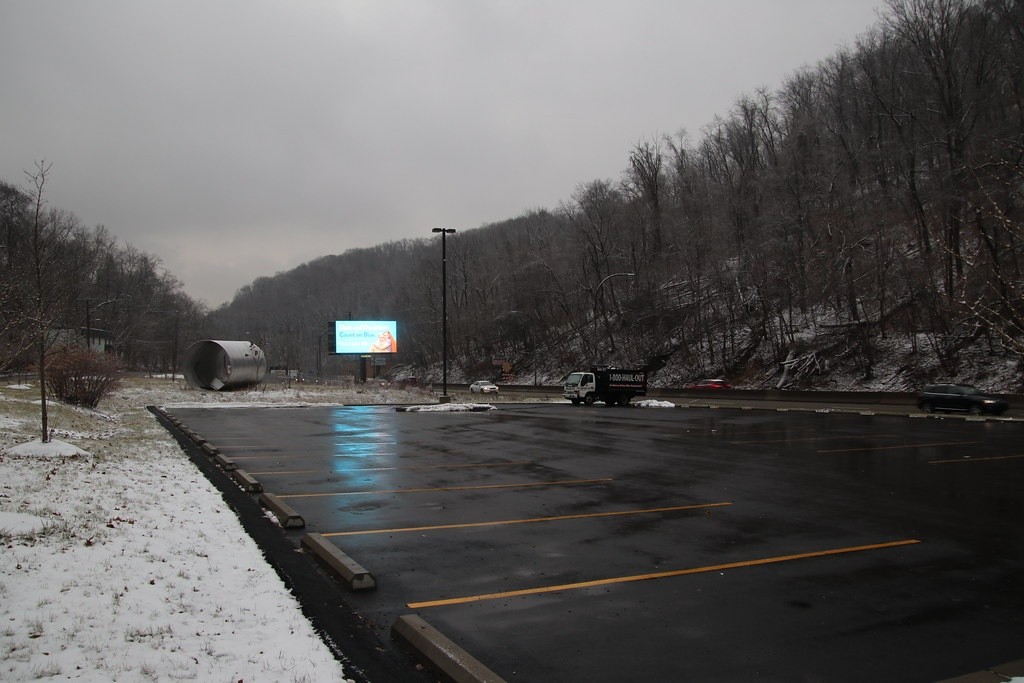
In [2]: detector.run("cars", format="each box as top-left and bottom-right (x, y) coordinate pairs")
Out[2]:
(470, 381), (499, 394)
(690, 379), (732, 389)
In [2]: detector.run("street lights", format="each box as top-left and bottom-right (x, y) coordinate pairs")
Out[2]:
(594, 273), (636, 363)
(433, 227), (457, 394)
(512, 311), (536, 386)
(246, 332), (267, 363)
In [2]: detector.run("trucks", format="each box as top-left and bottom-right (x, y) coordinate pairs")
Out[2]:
(564, 365), (647, 406)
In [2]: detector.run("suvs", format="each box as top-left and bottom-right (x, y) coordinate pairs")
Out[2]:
(917, 384), (1010, 416)
